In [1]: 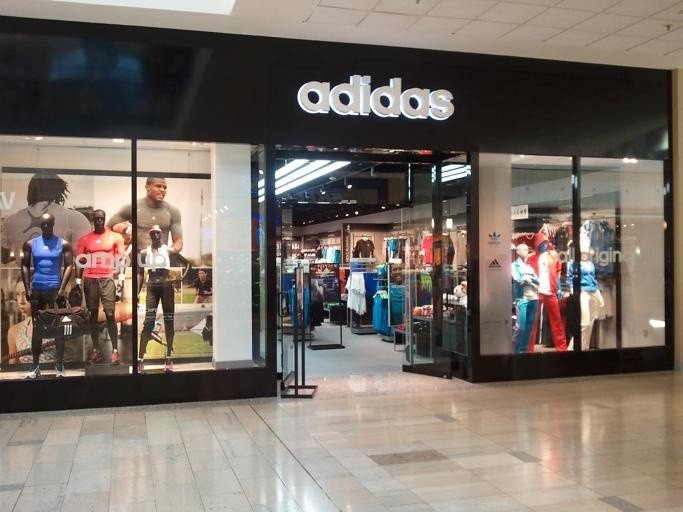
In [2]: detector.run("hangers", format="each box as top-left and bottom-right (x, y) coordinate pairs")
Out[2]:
(371, 289), (389, 299)
(538, 218), (611, 236)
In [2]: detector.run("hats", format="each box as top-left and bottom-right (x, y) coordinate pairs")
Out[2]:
(149, 225), (162, 235)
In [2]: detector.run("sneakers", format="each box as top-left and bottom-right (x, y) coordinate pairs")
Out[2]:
(137, 360), (144, 374)
(164, 358), (173, 372)
(89, 351), (103, 364)
(55, 365), (65, 378)
(112, 351), (119, 362)
(25, 365), (40, 379)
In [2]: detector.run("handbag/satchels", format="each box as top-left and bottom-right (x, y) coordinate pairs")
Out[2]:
(35, 307), (93, 339)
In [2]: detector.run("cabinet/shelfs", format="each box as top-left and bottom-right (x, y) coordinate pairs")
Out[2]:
(392, 301), (466, 358)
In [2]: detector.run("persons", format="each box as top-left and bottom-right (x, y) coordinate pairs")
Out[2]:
(297, 270), (303, 308)
(0, 172), (184, 379)
(192, 268), (212, 303)
(510, 232), (602, 353)
(390, 263), (404, 327)
